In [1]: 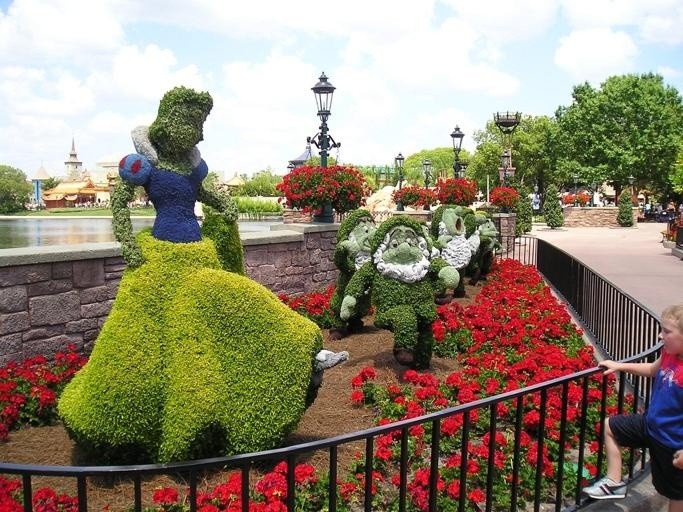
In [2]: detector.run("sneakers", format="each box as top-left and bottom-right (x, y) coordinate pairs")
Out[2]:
(583, 477), (626, 500)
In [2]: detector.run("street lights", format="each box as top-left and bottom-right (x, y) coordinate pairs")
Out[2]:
(628, 174), (634, 196)
(395, 155), (405, 211)
(573, 176), (579, 206)
(533, 184), (538, 206)
(450, 126), (464, 178)
(311, 72), (340, 223)
(423, 159), (433, 210)
(501, 150), (510, 180)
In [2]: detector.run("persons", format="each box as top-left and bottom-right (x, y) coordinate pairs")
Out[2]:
(582, 304), (683, 512)
(641, 199), (676, 221)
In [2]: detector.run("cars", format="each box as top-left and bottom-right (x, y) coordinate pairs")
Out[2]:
(645, 207), (676, 222)
